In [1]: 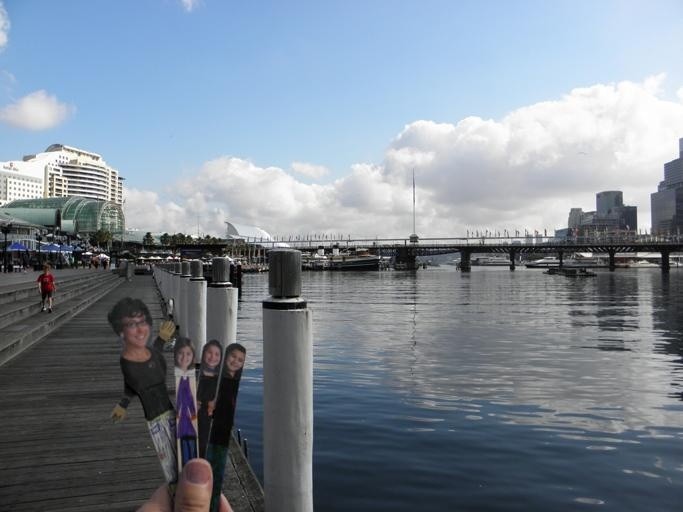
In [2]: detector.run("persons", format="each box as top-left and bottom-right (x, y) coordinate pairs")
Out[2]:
(37, 262), (57, 314)
(108, 297), (246, 512)
(87, 254), (108, 270)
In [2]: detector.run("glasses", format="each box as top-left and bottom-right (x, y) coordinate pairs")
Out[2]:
(124, 317), (149, 329)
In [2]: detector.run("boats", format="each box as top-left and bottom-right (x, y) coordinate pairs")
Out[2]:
(563, 258), (604, 267)
(302, 244), (392, 272)
(629, 260), (659, 268)
(472, 256), (511, 265)
(670, 261), (681, 267)
(525, 257), (560, 268)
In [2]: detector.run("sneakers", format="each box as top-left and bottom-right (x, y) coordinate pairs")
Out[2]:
(42, 307), (47, 311)
(48, 308), (52, 313)
